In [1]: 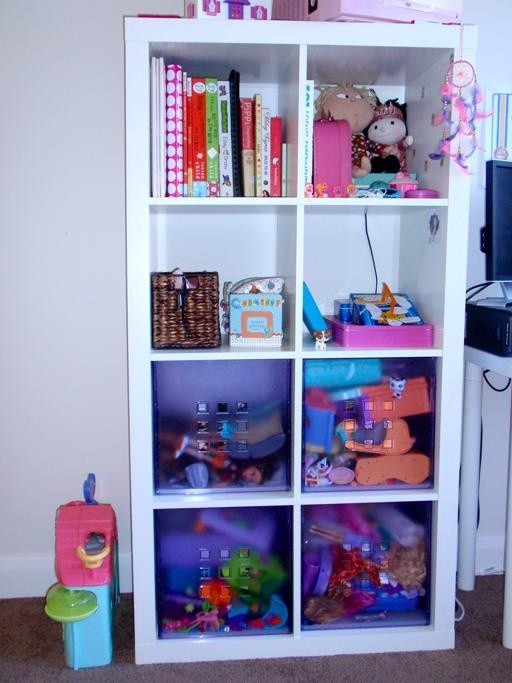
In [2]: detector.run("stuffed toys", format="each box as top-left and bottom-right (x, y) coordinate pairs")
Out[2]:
(314, 77), (398, 176)
(368, 87), (414, 171)
(313, 328), (331, 350)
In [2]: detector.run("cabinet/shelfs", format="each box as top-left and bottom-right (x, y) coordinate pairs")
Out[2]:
(123, 17), (477, 666)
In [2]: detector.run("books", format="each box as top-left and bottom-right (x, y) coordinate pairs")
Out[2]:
(302, 281), (334, 340)
(151, 52), (286, 202)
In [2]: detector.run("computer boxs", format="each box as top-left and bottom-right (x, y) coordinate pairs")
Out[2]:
(464, 301), (512, 357)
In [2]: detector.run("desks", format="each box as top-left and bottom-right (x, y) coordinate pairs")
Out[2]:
(457, 336), (512, 650)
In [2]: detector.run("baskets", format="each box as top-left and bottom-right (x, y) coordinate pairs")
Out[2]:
(151, 272), (221, 349)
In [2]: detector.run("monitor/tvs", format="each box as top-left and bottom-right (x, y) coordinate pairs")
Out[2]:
(476, 160), (512, 307)
